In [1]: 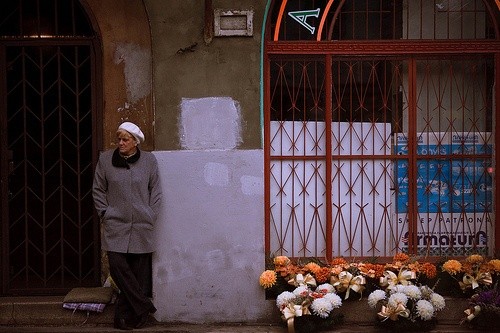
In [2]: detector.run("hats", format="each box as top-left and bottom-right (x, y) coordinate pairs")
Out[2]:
(119, 122), (145, 144)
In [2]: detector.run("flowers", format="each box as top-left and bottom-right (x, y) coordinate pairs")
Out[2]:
(259, 252), (500, 321)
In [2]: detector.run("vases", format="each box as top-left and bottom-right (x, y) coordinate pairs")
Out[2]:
(288, 316), (333, 333)
(381, 317), (432, 333)
(470, 314), (500, 333)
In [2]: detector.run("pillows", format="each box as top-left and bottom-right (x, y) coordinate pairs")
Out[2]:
(63, 287), (113, 304)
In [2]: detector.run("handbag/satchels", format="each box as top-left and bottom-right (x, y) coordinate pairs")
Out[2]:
(63, 287), (113, 327)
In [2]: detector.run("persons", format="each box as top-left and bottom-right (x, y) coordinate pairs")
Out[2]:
(92, 122), (162, 330)
